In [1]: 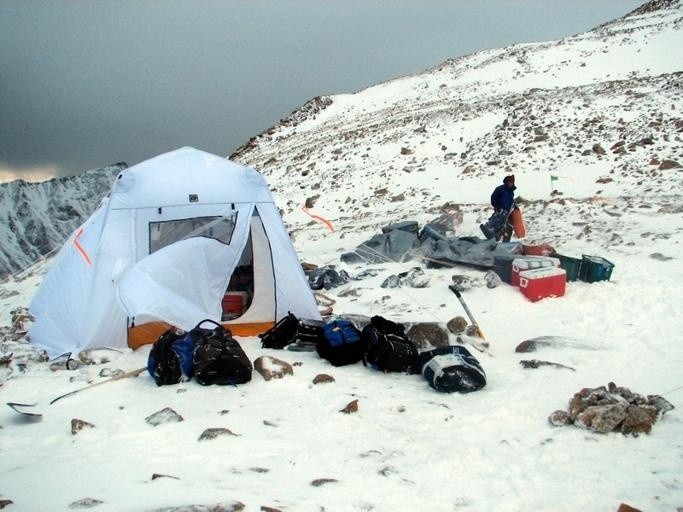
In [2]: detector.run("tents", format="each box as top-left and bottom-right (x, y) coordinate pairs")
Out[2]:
(24, 144), (324, 359)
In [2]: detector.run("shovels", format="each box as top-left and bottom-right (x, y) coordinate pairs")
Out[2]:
(6, 367), (148, 416)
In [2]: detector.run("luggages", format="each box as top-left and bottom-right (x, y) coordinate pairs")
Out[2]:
(296, 318), (326, 346)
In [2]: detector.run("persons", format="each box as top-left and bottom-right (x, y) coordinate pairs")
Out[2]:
(490, 174), (520, 243)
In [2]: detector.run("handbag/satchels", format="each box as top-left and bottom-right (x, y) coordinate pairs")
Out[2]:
(259, 311), (299, 349)
(419, 345), (486, 391)
(318, 321), (364, 366)
(363, 315), (418, 372)
(148, 317), (252, 386)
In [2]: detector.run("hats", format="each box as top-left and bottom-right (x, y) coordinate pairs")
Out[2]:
(503, 175), (514, 183)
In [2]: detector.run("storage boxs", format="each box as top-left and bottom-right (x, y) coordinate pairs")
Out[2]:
(512, 244), (616, 300)
(221, 290), (247, 314)
